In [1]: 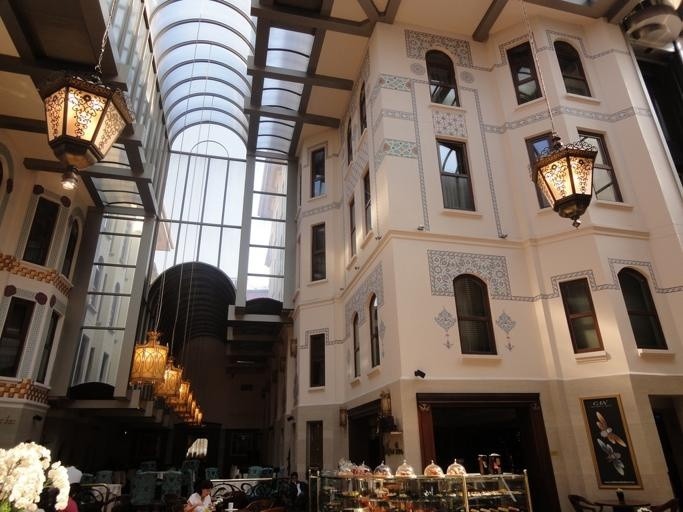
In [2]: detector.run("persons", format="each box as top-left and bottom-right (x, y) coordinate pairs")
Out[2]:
(39, 459), (317, 511)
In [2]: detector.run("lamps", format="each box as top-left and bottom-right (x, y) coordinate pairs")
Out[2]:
(519, 1), (599, 232)
(379, 392), (391, 417)
(39, 0), (135, 191)
(129, 0), (215, 428)
(289, 338), (298, 356)
(339, 408), (347, 426)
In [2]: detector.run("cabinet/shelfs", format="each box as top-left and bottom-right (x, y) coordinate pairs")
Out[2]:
(308, 468), (533, 512)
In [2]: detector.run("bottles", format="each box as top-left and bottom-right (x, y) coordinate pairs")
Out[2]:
(480, 454), (501, 475)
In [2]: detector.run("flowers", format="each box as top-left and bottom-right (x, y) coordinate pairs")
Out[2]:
(0, 441), (70, 512)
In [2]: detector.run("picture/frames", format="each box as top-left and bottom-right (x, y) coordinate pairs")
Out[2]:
(576, 393), (644, 490)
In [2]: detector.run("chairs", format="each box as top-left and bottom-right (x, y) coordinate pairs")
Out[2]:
(650, 496), (683, 512)
(567, 494), (604, 512)
(36, 460), (307, 512)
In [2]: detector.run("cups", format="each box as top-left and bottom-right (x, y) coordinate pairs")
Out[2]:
(243, 473), (249, 478)
(229, 502), (233, 510)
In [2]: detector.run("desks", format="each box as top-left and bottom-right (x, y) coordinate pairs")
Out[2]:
(592, 499), (651, 512)
